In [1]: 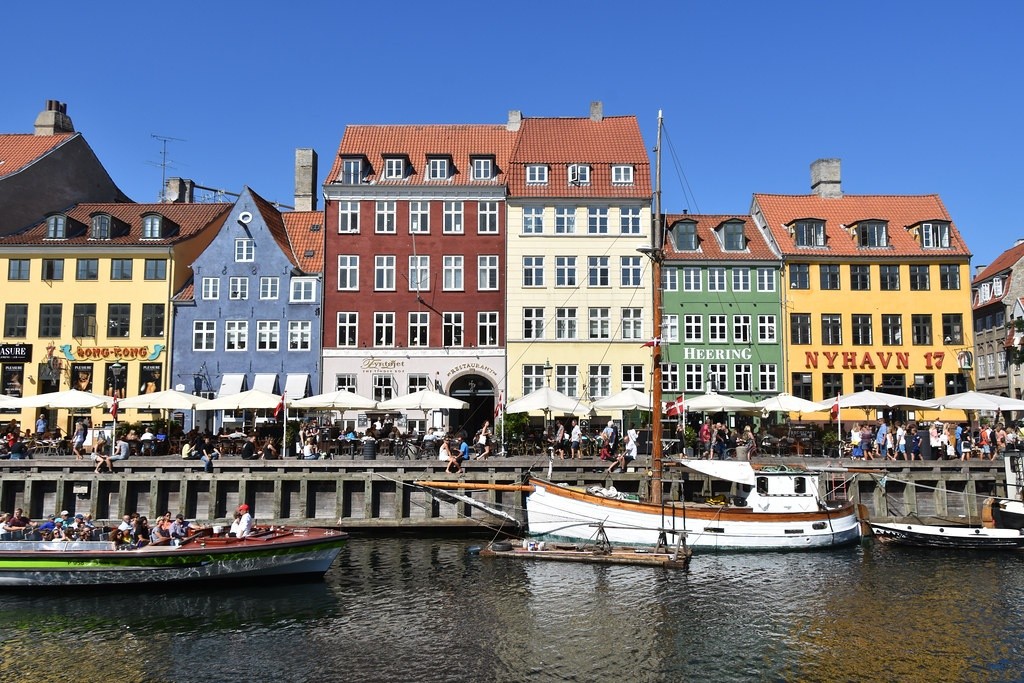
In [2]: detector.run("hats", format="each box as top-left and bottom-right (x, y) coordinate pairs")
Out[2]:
(48, 514), (55, 520)
(54, 517), (64, 523)
(61, 510), (69, 516)
(176, 514), (183, 519)
(239, 505), (249, 511)
(75, 514), (83, 518)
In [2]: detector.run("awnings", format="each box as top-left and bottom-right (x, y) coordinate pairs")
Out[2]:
(218, 374), (309, 400)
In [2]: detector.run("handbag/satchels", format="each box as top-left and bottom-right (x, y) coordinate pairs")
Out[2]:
(478, 434), (486, 445)
(964, 442), (971, 448)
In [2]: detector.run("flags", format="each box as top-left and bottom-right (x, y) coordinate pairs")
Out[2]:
(666, 395), (683, 417)
(107, 394), (118, 419)
(831, 397), (838, 420)
(494, 395), (502, 418)
(274, 395), (285, 419)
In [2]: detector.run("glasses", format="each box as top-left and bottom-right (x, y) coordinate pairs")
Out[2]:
(133, 516), (140, 519)
(54, 532), (59, 535)
(313, 421), (318, 424)
(240, 518), (241, 520)
(458, 439), (462, 442)
(79, 533), (86, 537)
(86, 530), (91, 532)
(445, 440), (451, 442)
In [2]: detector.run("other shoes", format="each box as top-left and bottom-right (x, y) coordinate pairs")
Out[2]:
(76, 456), (83, 460)
(204, 469), (210, 473)
(446, 469), (452, 473)
(106, 471), (113, 473)
(477, 457), (480, 461)
(456, 470), (463, 473)
(94, 469), (100, 474)
(619, 469), (626, 473)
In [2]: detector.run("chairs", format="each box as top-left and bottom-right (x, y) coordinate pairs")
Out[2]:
(472, 438), (624, 457)
(33, 437), (65, 456)
(126, 436), (437, 456)
(762, 435), (813, 457)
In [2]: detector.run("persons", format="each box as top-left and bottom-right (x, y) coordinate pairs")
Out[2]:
(91, 427), (279, 473)
(299, 414), (437, 460)
(699, 419), (788, 462)
(70, 418), (88, 460)
(0, 413), (61, 459)
(230, 504), (252, 537)
(547, 420), (640, 473)
(0, 508), (205, 547)
(424, 420), (492, 473)
(849, 418), (1024, 460)
(676, 424), (688, 459)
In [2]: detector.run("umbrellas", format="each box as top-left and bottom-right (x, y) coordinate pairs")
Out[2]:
(0, 388), (281, 435)
(505, 386), (667, 429)
(684, 390), (1024, 430)
(290, 388), (469, 434)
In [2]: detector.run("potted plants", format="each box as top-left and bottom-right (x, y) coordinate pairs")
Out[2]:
(681, 426), (696, 458)
(822, 432), (840, 457)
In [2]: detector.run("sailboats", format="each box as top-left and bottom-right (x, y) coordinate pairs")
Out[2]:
(413, 108), (861, 553)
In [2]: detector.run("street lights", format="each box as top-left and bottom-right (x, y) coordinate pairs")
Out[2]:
(112, 361), (122, 456)
(543, 359), (554, 426)
(962, 360), (975, 435)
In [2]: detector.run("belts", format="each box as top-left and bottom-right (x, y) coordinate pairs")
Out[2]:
(304, 454), (313, 457)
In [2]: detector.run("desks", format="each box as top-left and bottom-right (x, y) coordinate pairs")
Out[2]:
(661, 439), (680, 460)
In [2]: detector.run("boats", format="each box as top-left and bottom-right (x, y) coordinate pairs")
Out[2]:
(479, 524), (694, 568)
(0, 527), (347, 589)
(862, 473), (1024, 551)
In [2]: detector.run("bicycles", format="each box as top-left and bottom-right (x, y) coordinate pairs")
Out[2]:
(723, 436), (781, 473)
(394, 440), (438, 460)
